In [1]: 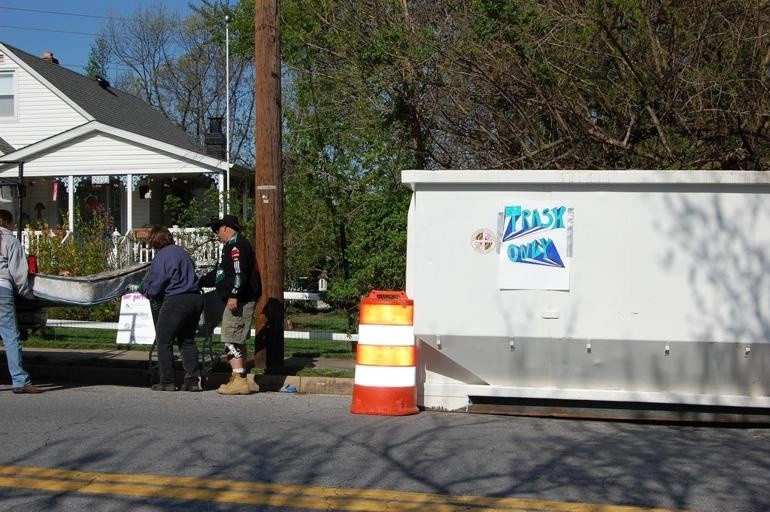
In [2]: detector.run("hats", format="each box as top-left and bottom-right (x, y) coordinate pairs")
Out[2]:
(211, 215), (243, 232)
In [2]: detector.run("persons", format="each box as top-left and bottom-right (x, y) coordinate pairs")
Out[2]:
(0, 209), (43, 393)
(128, 223), (203, 392)
(199, 215), (262, 395)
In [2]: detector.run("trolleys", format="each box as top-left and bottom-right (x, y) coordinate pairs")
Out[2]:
(144, 288), (224, 389)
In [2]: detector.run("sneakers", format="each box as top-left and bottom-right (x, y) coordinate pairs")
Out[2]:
(13, 385), (41, 394)
(180, 383), (199, 391)
(151, 382), (176, 390)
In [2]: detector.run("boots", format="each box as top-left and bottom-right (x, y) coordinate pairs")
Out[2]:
(218, 372), (250, 395)
